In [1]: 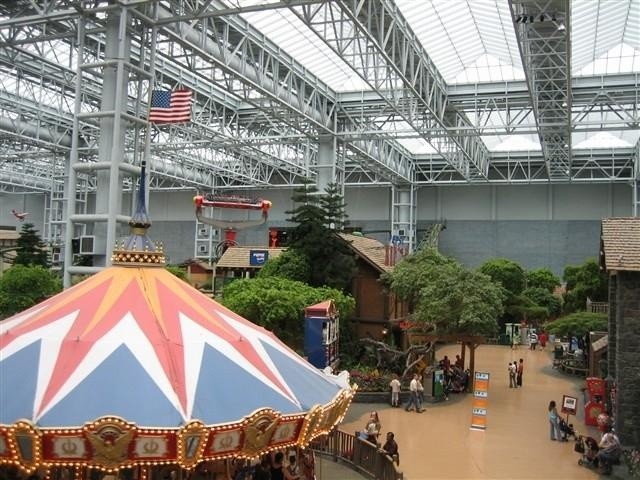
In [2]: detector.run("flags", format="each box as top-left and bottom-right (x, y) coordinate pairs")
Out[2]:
(148, 89), (193, 125)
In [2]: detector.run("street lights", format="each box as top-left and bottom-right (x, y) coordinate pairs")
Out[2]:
(510, 322), (515, 348)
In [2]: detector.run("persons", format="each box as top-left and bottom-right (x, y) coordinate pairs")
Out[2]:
(578, 335), (585, 349)
(568, 423), (574, 432)
(595, 434), (622, 475)
(548, 400), (566, 442)
(516, 358), (524, 387)
(600, 427), (620, 445)
(509, 360), (518, 388)
(530, 331), (538, 351)
(538, 330), (547, 352)
(508, 363), (513, 371)
(390, 354), (470, 414)
(232, 409), (400, 479)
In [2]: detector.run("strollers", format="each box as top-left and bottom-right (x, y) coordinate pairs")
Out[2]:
(557, 417), (578, 443)
(573, 436), (603, 468)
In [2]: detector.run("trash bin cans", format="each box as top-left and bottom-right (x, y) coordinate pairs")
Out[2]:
(555, 345), (563, 358)
(500, 334), (510, 344)
